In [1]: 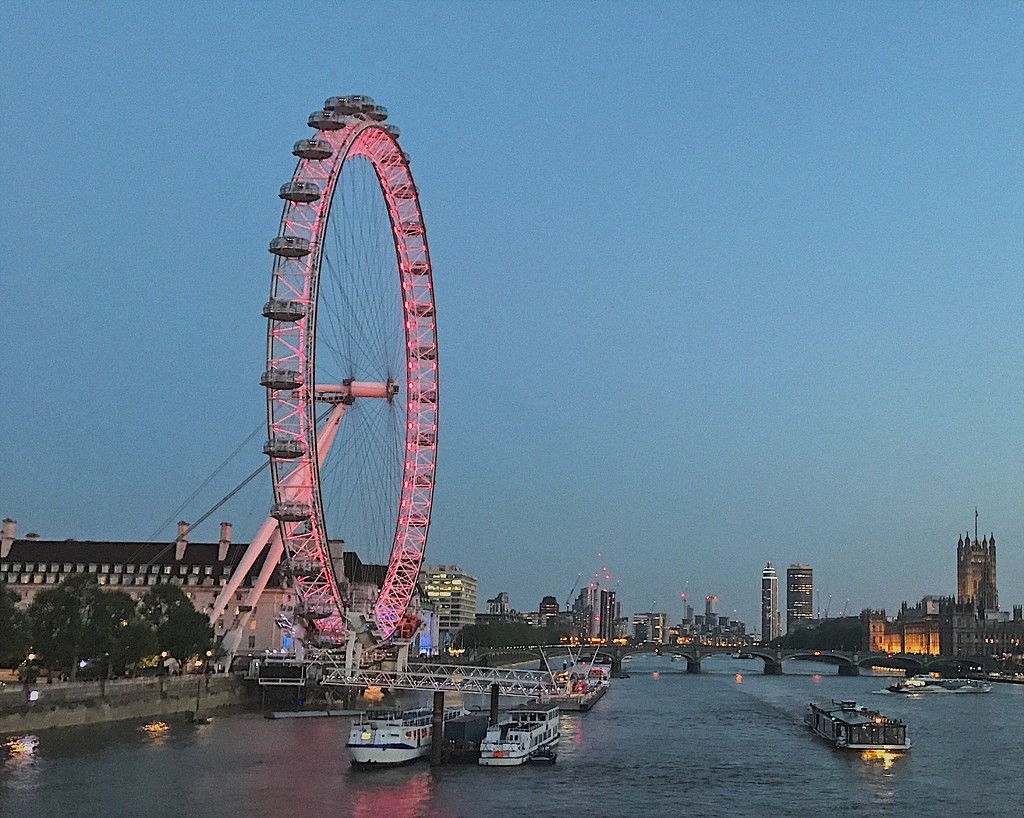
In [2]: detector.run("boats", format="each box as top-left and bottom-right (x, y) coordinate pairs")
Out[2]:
(476, 698), (561, 765)
(885, 673), (994, 695)
(441, 737), (479, 767)
(344, 705), (464, 770)
(622, 656), (633, 663)
(619, 674), (630, 679)
(531, 744), (557, 767)
(803, 698), (912, 754)
(671, 654), (681, 662)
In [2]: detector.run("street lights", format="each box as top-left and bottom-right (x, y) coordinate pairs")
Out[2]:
(159, 651), (167, 693)
(25, 647), (38, 690)
(206, 651), (212, 676)
